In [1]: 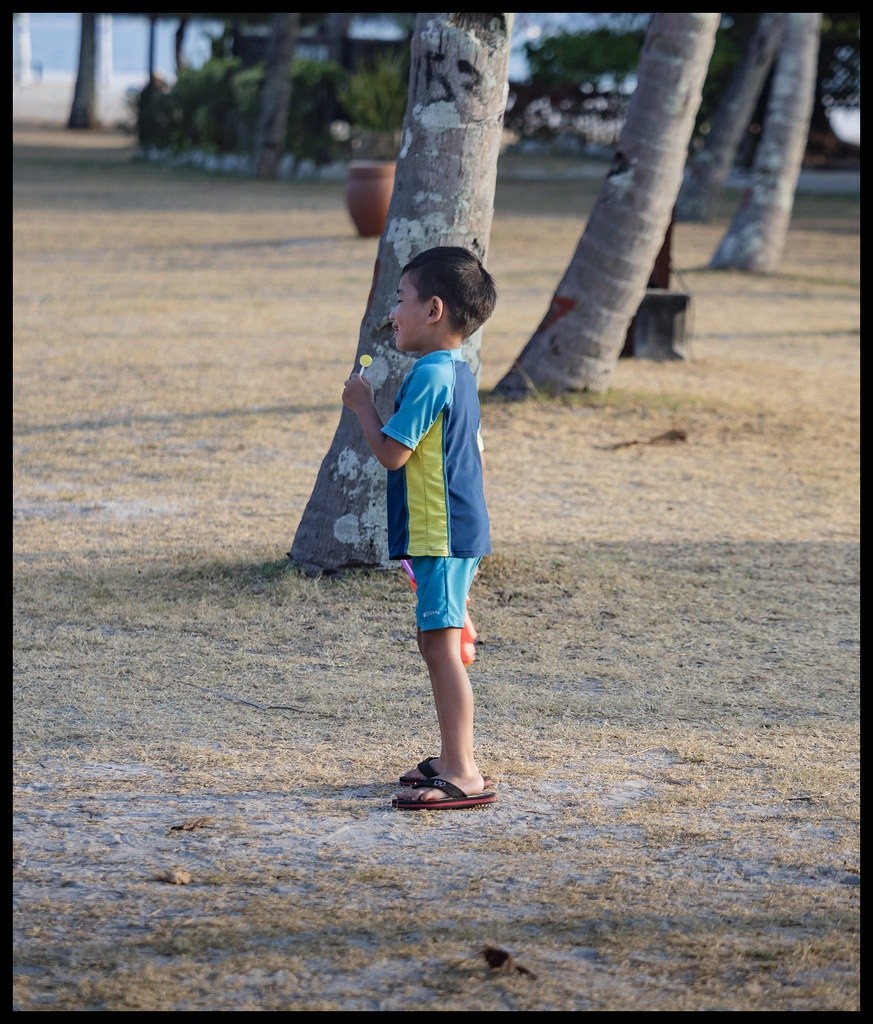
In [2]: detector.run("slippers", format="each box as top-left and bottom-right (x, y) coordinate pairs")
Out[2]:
(391, 780), (497, 809)
(400, 758), (442, 786)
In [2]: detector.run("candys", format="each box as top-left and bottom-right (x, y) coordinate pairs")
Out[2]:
(359, 354), (372, 368)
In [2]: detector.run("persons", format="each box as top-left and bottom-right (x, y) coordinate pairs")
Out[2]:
(343, 244), (499, 812)
